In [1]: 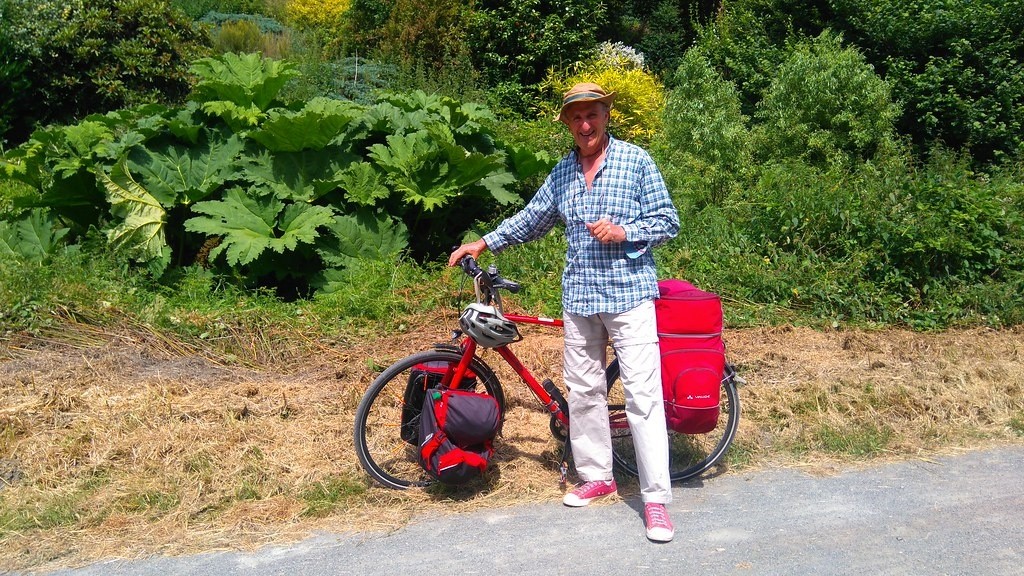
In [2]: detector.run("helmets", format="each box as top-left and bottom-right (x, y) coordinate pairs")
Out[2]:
(459, 303), (523, 347)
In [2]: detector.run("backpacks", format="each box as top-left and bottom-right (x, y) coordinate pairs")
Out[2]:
(656, 280), (725, 434)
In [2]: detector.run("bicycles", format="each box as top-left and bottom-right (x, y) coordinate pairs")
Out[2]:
(354, 246), (747, 494)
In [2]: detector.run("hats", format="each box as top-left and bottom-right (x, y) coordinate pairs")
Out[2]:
(553, 82), (617, 124)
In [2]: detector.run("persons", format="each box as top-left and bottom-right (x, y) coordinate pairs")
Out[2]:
(449, 83), (680, 542)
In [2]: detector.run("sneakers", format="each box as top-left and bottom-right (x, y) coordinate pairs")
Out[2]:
(563, 477), (617, 507)
(645, 501), (673, 542)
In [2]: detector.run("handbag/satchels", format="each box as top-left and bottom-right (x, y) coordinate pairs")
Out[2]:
(417, 387), (500, 483)
(400, 358), (477, 447)
